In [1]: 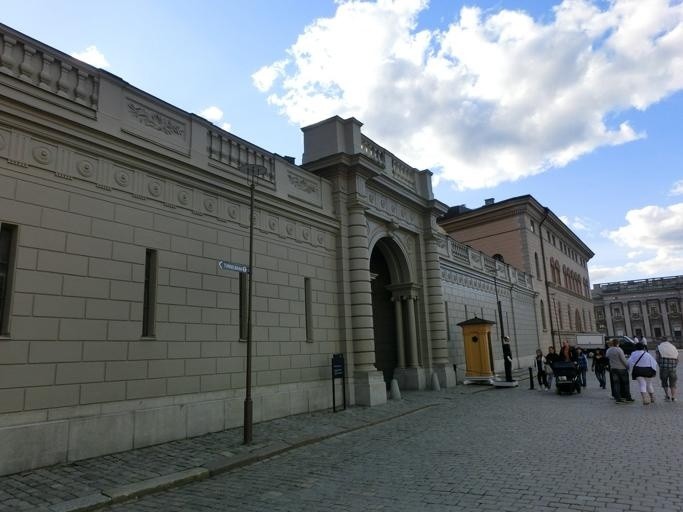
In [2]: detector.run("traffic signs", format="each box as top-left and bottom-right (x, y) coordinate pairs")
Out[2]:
(219, 261), (248, 272)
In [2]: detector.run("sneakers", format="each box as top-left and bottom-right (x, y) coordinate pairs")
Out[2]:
(610, 395), (677, 405)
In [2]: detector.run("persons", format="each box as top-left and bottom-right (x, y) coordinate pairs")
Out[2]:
(604, 339), (635, 404)
(500, 336), (515, 382)
(639, 336), (648, 353)
(590, 347), (607, 389)
(530, 349), (549, 393)
(626, 342), (657, 405)
(654, 335), (679, 401)
(632, 335), (638, 344)
(558, 339), (578, 366)
(544, 345), (559, 389)
(574, 345), (588, 388)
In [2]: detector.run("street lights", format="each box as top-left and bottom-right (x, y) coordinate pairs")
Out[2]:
(551, 292), (562, 348)
(237, 163), (267, 444)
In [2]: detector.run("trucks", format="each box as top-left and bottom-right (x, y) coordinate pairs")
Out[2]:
(575, 334), (636, 357)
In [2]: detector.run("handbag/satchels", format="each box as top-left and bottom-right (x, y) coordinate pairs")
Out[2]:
(632, 367), (636, 380)
